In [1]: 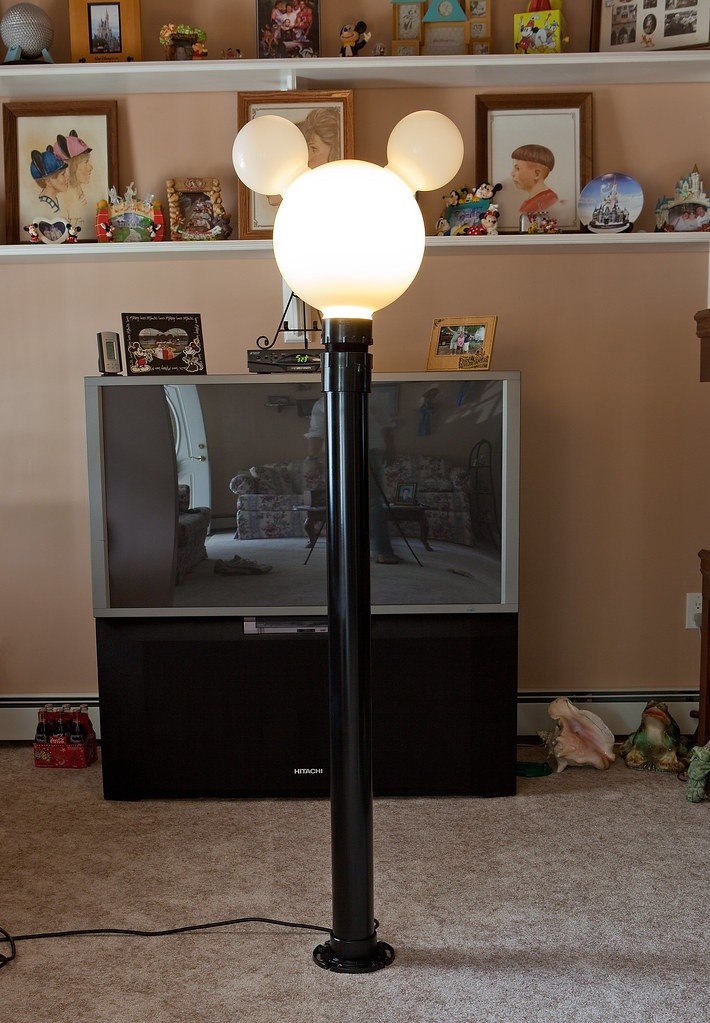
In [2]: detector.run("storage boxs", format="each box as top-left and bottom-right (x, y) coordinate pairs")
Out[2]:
(513, 10), (565, 53)
(33, 711), (99, 769)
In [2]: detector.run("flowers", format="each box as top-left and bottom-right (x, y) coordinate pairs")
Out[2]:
(159, 25), (206, 44)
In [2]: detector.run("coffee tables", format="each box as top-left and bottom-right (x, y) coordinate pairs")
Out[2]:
(288, 506), (435, 555)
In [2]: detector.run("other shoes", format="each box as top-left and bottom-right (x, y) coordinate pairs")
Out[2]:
(215, 554), (273, 575)
(376, 553), (399, 565)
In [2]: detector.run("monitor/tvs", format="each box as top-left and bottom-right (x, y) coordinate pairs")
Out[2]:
(83, 369), (520, 618)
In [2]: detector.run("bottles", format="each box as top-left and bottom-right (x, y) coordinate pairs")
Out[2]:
(34, 701), (94, 745)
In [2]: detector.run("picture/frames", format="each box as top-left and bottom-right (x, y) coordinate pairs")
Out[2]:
(468, 41), (490, 55)
(391, 39), (419, 56)
(236, 88), (355, 238)
(393, 483), (418, 506)
(426, 316), (497, 371)
(69, 1), (142, 62)
(166, 33), (198, 62)
(0, 101), (119, 243)
(120, 312), (208, 376)
(476, 92), (595, 235)
(165, 175), (223, 241)
(465, 0), (490, 20)
(470, 20), (490, 40)
(391, 0), (424, 46)
(589, 0), (710, 52)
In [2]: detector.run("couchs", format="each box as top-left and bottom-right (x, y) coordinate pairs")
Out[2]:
(174, 485), (211, 585)
(230, 460), (328, 539)
(375, 450), (473, 541)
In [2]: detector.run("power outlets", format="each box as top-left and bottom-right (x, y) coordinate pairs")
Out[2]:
(686, 592), (702, 630)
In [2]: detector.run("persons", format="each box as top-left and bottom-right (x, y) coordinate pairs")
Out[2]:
(304, 389), (398, 564)
(270, 0), (313, 48)
(43, 225), (62, 241)
(447, 327), (473, 356)
(154, 344), (174, 360)
(671, 206), (710, 231)
(398, 488), (412, 503)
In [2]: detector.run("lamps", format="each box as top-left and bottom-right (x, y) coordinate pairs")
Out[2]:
(229, 108), (466, 972)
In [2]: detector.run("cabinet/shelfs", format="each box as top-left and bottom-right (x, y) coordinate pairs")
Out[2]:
(95, 613), (518, 802)
(0, 50), (710, 266)
